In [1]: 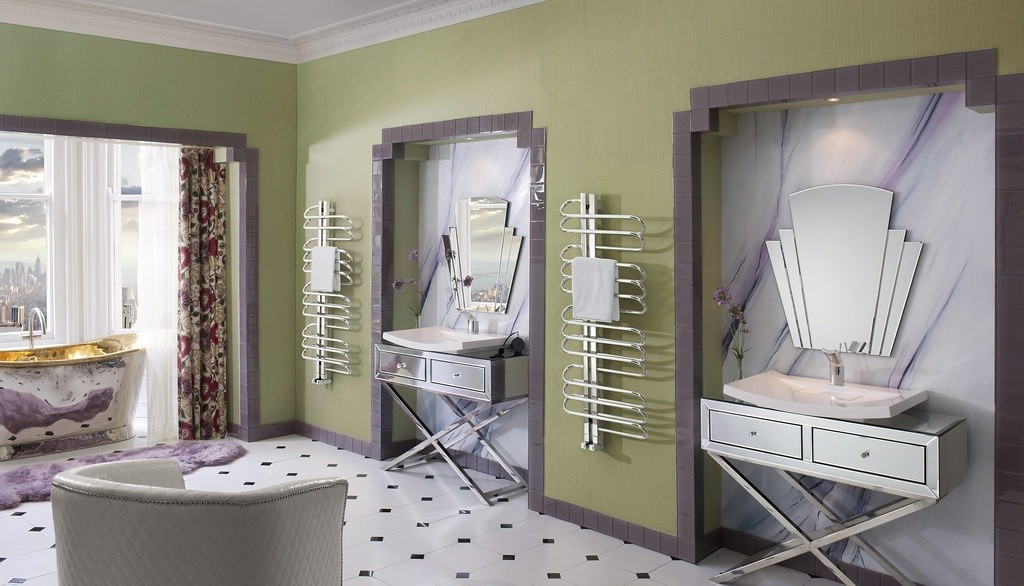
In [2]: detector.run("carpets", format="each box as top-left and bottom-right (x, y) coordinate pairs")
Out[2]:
(0, 439), (246, 509)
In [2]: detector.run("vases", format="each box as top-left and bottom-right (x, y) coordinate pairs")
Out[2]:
(412, 315), (422, 329)
(733, 358), (743, 405)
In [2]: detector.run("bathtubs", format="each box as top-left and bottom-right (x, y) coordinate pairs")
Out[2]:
(0, 332), (149, 461)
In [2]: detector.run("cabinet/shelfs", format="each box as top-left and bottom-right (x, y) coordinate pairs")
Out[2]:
(372, 342), (529, 506)
(699, 393), (970, 586)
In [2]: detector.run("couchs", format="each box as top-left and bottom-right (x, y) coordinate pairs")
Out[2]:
(50, 454), (348, 586)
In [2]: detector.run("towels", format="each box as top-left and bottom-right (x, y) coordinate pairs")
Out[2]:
(309, 244), (342, 294)
(568, 254), (625, 325)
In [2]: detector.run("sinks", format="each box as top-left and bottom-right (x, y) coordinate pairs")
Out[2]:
(722, 367), (933, 422)
(381, 323), (520, 356)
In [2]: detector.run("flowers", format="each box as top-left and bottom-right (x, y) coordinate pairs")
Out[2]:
(391, 248), (424, 328)
(712, 285), (754, 379)
(446, 249), (474, 299)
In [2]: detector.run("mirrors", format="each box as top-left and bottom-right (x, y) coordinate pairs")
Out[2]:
(441, 196), (525, 314)
(764, 183), (924, 357)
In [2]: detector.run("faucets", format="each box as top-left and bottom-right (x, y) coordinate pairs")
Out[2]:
(22, 307), (48, 349)
(453, 307), (483, 334)
(845, 337), (871, 354)
(821, 345), (847, 388)
